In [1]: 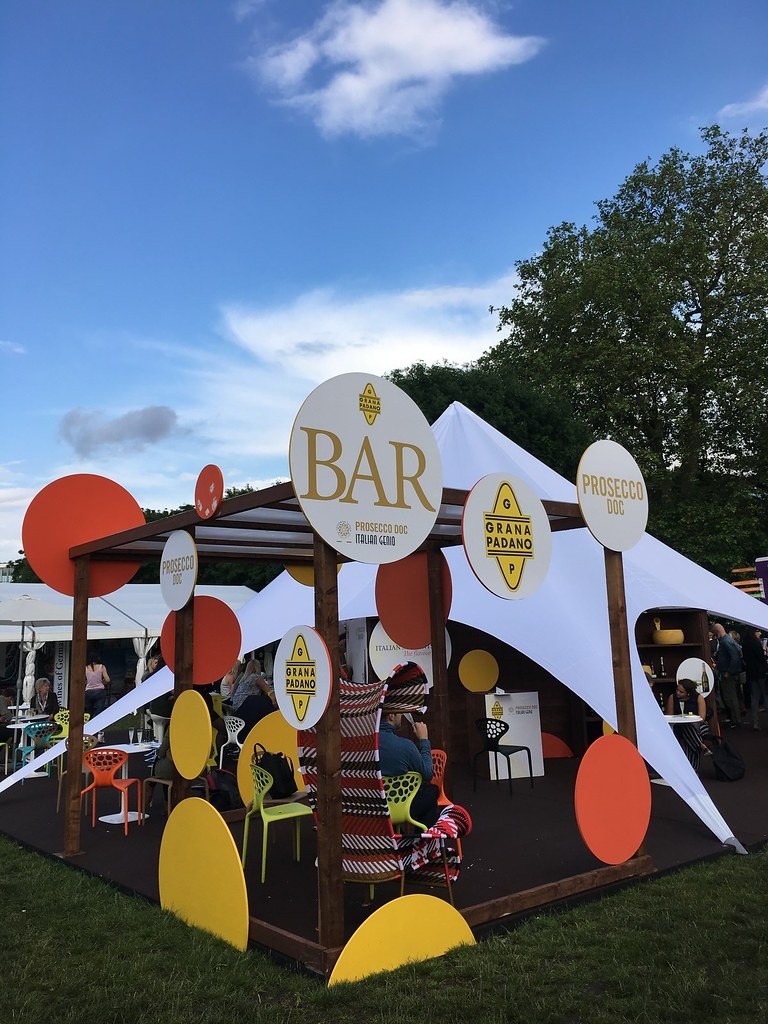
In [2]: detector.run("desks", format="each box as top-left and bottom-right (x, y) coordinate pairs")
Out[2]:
(649, 714), (703, 786)
(5, 722), (61, 778)
(7, 706), (30, 716)
(10, 715), (49, 723)
(88, 743), (155, 825)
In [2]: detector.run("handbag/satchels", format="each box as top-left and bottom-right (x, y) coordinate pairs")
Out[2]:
(254, 743), (298, 800)
(711, 735), (744, 782)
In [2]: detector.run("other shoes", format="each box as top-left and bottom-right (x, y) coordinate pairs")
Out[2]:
(98, 734), (106, 743)
(702, 748), (712, 759)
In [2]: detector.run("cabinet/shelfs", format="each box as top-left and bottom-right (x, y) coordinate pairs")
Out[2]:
(573, 607), (721, 750)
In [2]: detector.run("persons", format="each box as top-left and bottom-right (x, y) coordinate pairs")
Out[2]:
(659, 615), (768, 785)
(24, 642), (462, 889)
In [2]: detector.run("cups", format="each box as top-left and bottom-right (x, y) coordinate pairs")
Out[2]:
(28, 709), (34, 715)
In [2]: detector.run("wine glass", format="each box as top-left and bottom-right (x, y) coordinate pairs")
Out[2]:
(403, 712), (414, 725)
(129, 727), (134, 746)
(137, 729), (142, 746)
(680, 702), (685, 718)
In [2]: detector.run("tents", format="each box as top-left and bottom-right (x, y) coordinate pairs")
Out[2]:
(2, 401), (768, 862)
(102, 582), (261, 740)
(1, 566), (144, 776)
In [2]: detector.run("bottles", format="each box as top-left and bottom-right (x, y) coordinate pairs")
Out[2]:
(709, 657), (716, 668)
(660, 693), (666, 712)
(702, 663), (709, 692)
(658, 657), (666, 678)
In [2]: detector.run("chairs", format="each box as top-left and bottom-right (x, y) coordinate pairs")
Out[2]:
(473, 718), (534, 796)
(0, 660), (472, 907)
